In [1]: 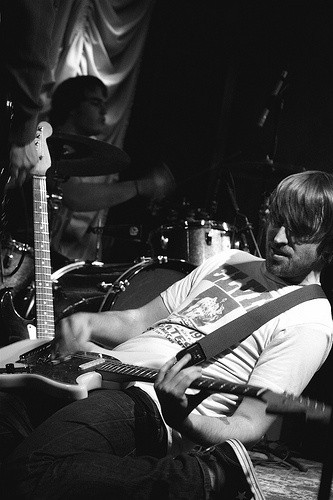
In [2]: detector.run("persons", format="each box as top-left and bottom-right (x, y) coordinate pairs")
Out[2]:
(46, 75), (171, 261)
(0, 169), (333, 500)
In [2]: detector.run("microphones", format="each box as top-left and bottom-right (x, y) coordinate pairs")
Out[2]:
(257, 70), (288, 127)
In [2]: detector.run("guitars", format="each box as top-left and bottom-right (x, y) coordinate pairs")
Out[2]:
(24, 121), (55, 339)
(0, 338), (332, 419)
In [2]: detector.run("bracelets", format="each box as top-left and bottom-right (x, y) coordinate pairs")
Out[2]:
(133, 179), (141, 198)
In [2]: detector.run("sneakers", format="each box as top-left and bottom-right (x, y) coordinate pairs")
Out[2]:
(203, 438), (264, 500)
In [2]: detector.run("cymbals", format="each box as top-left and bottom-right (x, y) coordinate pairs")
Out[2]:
(235, 162), (305, 180)
(48, 133), (131, 176)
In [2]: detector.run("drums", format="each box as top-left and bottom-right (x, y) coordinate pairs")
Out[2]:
(0, 241), (36, 291)
(26, 256), (199, 348)
(186, 220), (237, 265)
